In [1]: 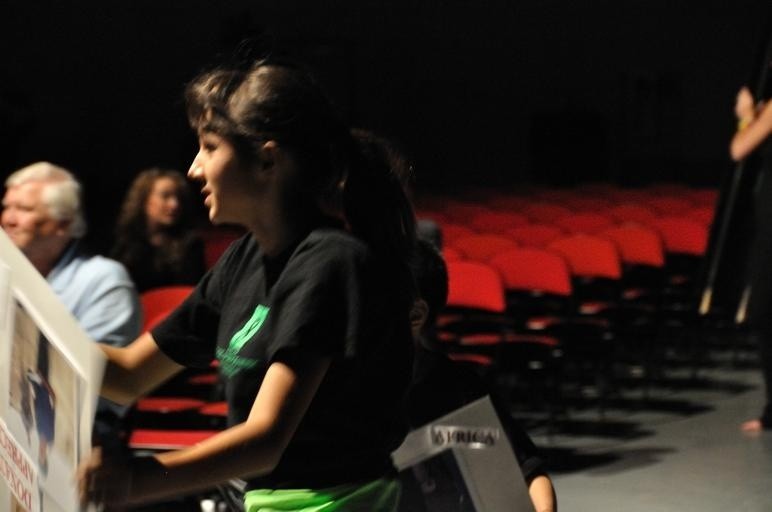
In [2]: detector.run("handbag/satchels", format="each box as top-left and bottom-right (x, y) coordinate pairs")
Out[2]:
(244, 472), (401, 511)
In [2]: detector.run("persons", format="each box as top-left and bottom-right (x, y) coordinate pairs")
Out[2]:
(694, 56), (770, 431)
(97, 55), (556, 512)
(1, 161), (141, 511)
